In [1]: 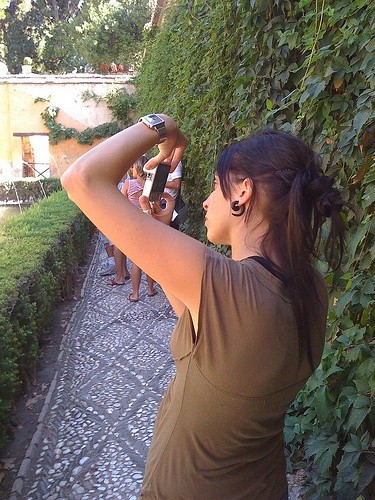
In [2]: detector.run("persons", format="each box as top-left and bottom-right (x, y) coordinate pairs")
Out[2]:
(98, 155), (182, 304)
(109, 60), (124, 74)
(61, 114), (360, 496)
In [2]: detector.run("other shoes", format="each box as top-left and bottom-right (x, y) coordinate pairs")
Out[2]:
(126, 259), (132, 267)
(97, 262), (115, 276)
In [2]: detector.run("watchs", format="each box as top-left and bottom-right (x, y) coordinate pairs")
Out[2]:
(137, 113), (170, 144)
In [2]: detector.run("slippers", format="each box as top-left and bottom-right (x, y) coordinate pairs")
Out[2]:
(106, 278), (125, 285)
(123, 274), (131, 280)
(146, 288), (157, 297)
(127, 293), (139, 302)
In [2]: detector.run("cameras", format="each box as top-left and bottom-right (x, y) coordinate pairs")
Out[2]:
(142, 162), (171, 202)
